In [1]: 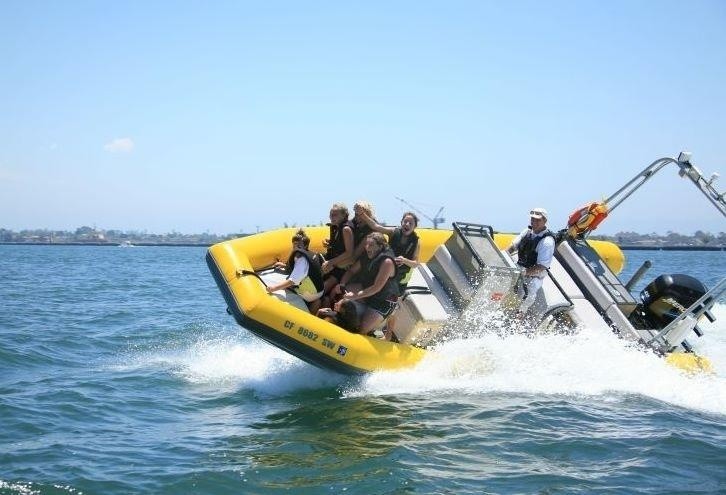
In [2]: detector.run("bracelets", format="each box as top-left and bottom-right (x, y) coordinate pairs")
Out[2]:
(359, 212), (365, 216)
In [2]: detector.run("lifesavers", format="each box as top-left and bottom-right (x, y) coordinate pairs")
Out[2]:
(567, 206), (588, 227)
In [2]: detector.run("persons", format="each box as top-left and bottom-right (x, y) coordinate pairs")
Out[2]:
(347, 232), (400, 334)
(338, 202), (380, 267)
(322, 204), (354, 293)
(316, 298), (356, 326)
(266, 231), (324, 315)
(354, 209), (421, 342)
(506, 208), (556, 317)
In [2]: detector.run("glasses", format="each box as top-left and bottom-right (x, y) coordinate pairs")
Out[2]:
(530, 211), (543, 215)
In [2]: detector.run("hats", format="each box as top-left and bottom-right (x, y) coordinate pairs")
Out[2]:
(530, 208), (548, 219)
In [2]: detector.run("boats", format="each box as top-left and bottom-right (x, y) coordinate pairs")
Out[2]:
(204, 150), (724, 386)
